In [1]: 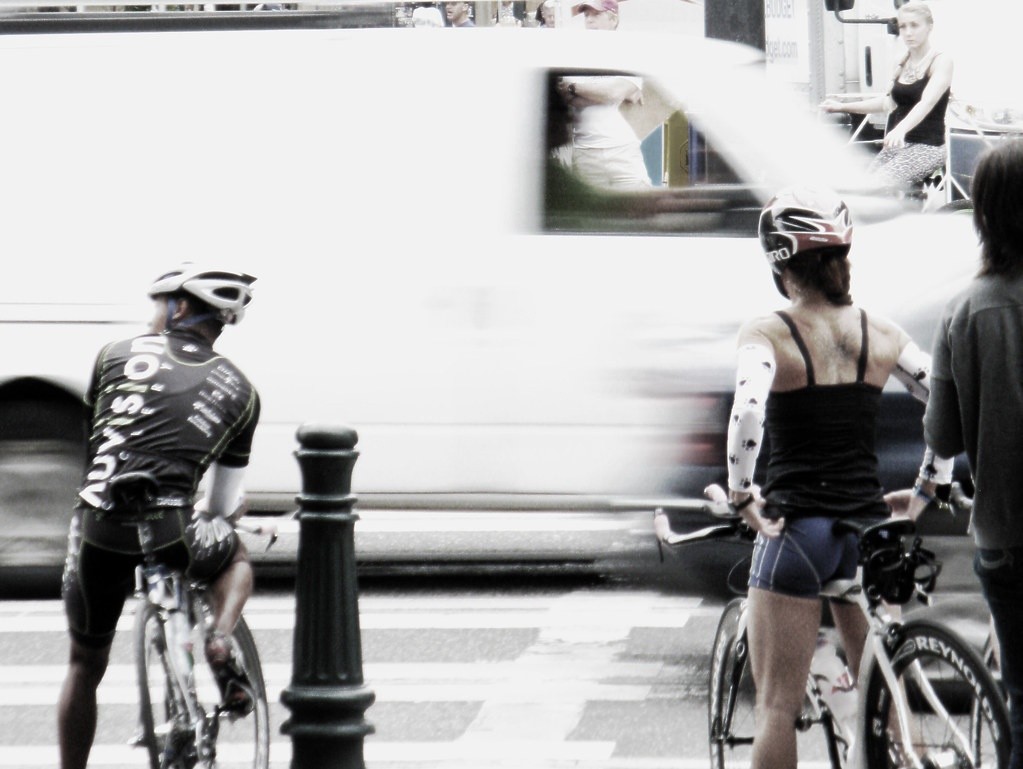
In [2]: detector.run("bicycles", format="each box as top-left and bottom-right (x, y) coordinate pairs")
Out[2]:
(130, 503), (273, 768)
(648, 478), (1017, 769)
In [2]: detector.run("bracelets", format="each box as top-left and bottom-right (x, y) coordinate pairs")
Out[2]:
(568, 82), (577, 95)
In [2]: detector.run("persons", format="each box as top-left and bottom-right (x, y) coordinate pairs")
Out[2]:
(922, 135), (1022, 721)
(411, 0), (558, 28)
(818, 1), (953, 189)
(559, 0), (651, 189)
(725, 190), (956, 769)
(53, 259), (262, 769)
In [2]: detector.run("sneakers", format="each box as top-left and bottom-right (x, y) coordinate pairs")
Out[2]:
(207, 632), (255, 718)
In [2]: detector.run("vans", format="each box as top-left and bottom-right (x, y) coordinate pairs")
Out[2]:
(0, 10), (986, 608)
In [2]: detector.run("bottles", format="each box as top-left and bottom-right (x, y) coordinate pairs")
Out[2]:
(172, 612), (194, 677)
(809, 632), (861, 743)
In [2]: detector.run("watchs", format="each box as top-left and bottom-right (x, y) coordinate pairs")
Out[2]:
(728, 494), (755, 515)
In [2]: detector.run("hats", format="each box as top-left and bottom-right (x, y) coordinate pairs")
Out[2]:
(576, 0), (619, 15)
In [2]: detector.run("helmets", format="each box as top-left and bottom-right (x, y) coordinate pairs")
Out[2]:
(149, 263), (259, 326)
(757, 187), (852, 254)
